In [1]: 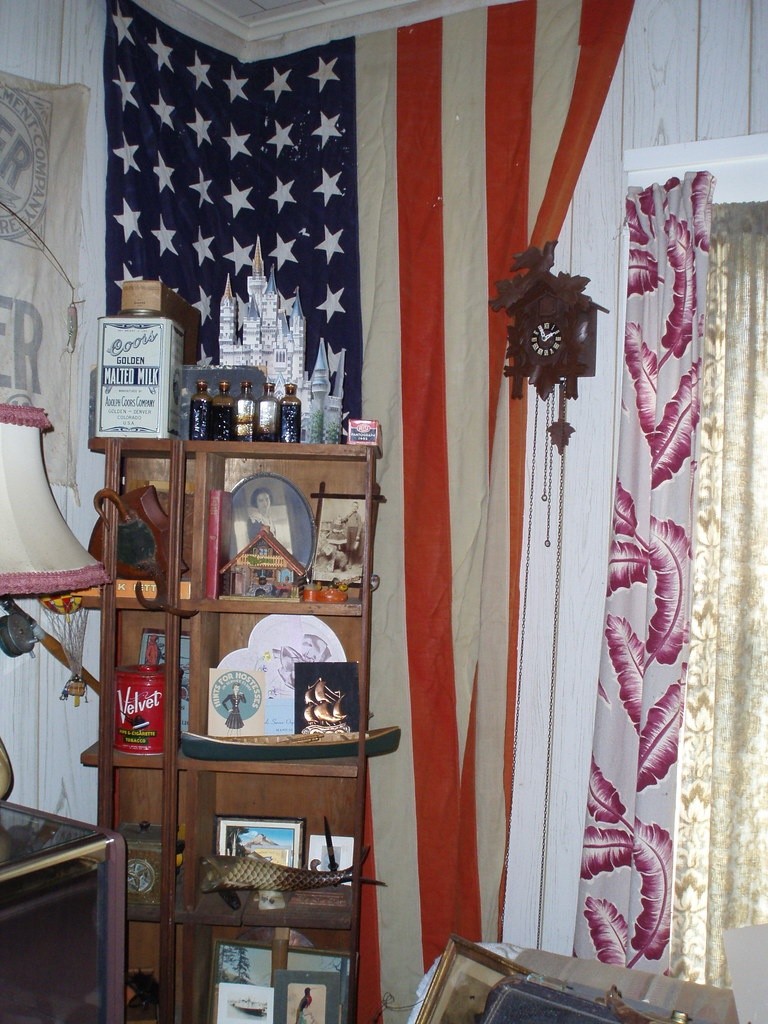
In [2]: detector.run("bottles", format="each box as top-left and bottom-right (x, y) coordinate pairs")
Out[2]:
(280, 384), (301, 443)
(211, 380), (235, 441)
(190, 381), (211, 440)
(256, 382), (281, 442)
(233, 381), (258, 442)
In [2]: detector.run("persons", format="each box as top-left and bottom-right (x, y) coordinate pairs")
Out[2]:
(246, 488), (277, 548)
(341, 501), (362, 570)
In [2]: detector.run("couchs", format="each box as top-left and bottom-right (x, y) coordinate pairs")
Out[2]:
(408, 942), (738, 1024)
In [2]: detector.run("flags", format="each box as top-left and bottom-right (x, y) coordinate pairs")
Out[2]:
(100, 0), (633, 1024)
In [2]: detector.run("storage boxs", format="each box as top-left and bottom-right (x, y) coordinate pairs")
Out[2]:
(121, 280), (201, 365)
(347, 418), (384, 459)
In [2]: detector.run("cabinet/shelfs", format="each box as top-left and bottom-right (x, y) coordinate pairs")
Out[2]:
(0, 800), (127, 1024)
(79, 438), (386, 1024)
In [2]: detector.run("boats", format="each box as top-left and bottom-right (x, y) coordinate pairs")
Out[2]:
(179, 725), (402, 762)
(228, 986), (268, 1014)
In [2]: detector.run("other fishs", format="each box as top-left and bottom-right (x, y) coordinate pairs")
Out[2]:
(199, 846), (388, 891)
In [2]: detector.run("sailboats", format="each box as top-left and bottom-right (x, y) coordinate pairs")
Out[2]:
(301, 677), (352, 733)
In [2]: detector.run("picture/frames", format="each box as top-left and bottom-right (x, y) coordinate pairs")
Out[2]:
(206, 939), (358, 1024)
(229, 472), (317, 573)
(310, 481), (387, 589)
(212, 813), (306, 870)
(415, 933), (562, 1024)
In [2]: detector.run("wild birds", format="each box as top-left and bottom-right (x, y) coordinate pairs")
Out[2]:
(295, 987), (312, 1024)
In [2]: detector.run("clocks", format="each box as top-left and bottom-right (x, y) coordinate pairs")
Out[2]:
(488, 240), (611, 456)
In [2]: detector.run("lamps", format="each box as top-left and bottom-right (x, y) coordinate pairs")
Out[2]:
(0, 402), (114, 800)
